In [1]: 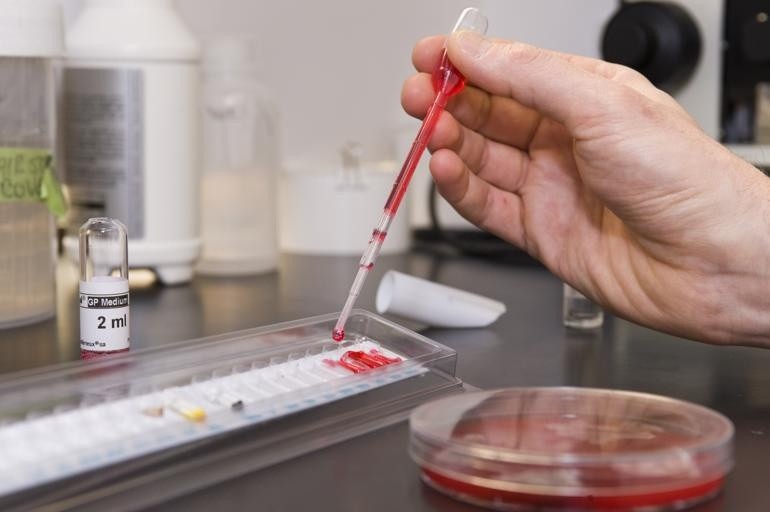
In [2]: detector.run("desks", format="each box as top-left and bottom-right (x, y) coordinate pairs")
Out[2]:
(0, 247), (770, 512)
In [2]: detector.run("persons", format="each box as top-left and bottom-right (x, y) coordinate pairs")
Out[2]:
(400, 34), (770, 350)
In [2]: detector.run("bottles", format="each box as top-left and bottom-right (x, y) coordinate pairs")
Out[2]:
(73, 213), (131, 377)
(0, 0), (59, 340)
(65, 0), (212, 286)
(562, 276), (605, 331)
(196, 28), (286, 280)
